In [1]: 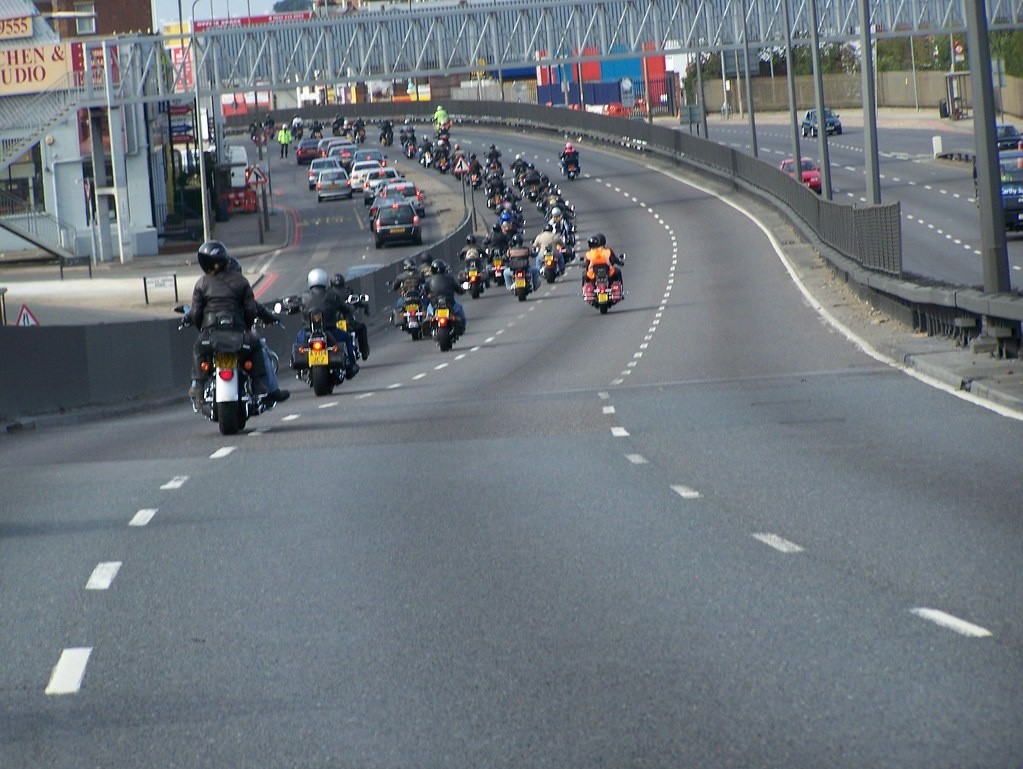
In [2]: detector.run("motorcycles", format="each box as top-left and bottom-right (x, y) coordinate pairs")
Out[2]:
(248, 110), (580, 354)
(173, 302), (284, 436)
(282, 290), (369, 398)
(431, 114), (452, 139)
(557, 150), (581, 182)
(579, 252), (627, 315)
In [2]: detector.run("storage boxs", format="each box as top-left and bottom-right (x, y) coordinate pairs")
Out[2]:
(291, 342), (309, 369)
(390, 307), (406, 327)
(611, 281), (621, 300)
(330, 341), (348, 366)
(583, 282), (596, 302)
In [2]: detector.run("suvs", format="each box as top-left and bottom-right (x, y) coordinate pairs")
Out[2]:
(351, 150), (388, 171)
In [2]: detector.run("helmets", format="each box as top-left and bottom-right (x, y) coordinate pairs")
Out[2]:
(512, 234), (523, 244)
(330, 274), (345, 287)
(308, 269), (328, 289)
(432, 259), (447, 273)
(227, 256), (243, 273)
(470, 143), (564, 222)
(467, 234), (476, 243)
(403, 258), (416, 269)
(588, 237), (599, 247)
(544, 224), (553, 232)
(259, 102), (461, 152)
(198, 241), (228, 273)
(596, 234), (606, 245)
(493, 224), (502, 232)
(421, 252), (432, 263)
(566, 143), (572, 149)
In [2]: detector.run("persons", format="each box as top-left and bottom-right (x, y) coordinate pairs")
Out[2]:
(805, 164), (812, 170)
(287, 269), (360, 381)
(182, 241), (290, 415)
(353, 116), (366, 140)
(458, 207), (577, 293)
(249, 112), (275, 144)
(582, 234), (625, 302)
(1000, 165), (1015, 181)
(380, 118), (465, 181)
(1003, 128), (1014, 137)
(329, 272), (370, 361)
(558, 143), (581, 178)
(332, 114), (352, 136)
(309, 121), (323, 139)
(389, 252), (467, 336)
(288, 114), (303, 139)
(465, 145), (567, 207)
(432, 105), (449, 137)
(278, 123), (292, 159)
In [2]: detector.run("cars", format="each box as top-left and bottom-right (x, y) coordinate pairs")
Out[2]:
(293, 138), (323, 165)
(378, 182), (427, 218)
(972, 123), (1023, 233)
(779, 156), (822, 193)
(368, 195), (413, 232)
(360, 167), (407, 205)
(315, 168), (354, 202)
(801, 107), (843, 139)
(307, 158), (344, 191)
(349, 160), (384, 192)
(371, 201), (424, 249)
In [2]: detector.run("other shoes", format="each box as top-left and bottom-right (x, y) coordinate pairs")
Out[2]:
(362, 351), (370, 360)
(270, 389), (290, 402)
(189, 383), (204, 396)
(346, 365), (359, 379)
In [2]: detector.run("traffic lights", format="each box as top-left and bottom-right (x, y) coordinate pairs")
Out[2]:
(954, 35), (962, 54)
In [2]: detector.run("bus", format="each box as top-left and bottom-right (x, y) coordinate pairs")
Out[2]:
(212, 145), (260, 216)
